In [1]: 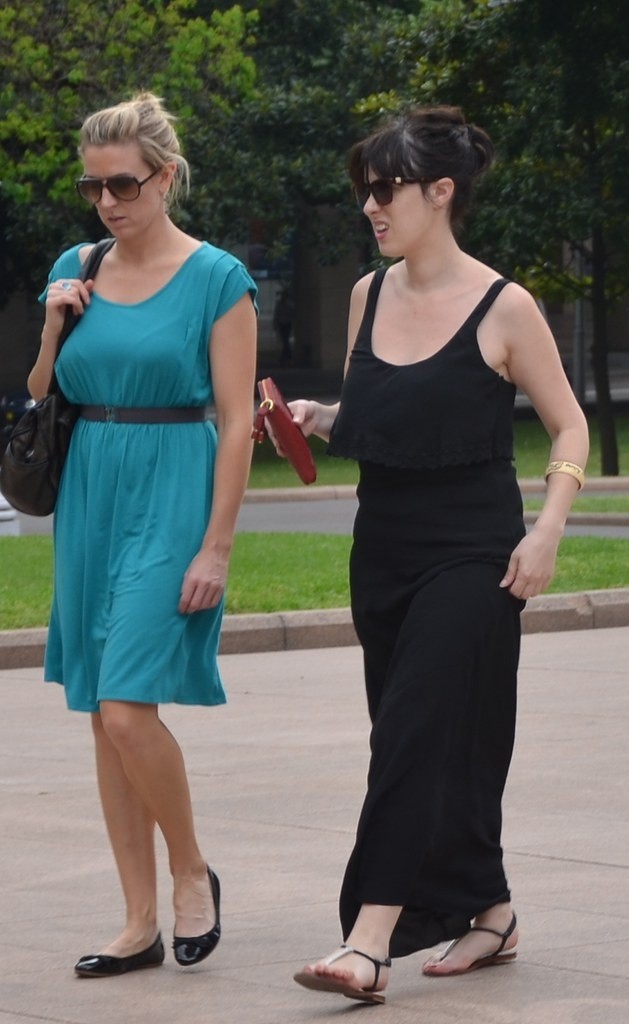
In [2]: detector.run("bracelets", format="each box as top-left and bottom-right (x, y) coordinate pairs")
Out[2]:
(544, 461), (585, 492)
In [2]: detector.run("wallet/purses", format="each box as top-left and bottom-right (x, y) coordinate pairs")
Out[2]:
(251, 376), (316, 485)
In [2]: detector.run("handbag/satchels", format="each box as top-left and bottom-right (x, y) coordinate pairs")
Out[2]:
(0, 236), (116, 518)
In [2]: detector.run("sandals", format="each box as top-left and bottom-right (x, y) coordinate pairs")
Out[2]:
(421, 909), (517, 975)
(293, 946), (391, 1004)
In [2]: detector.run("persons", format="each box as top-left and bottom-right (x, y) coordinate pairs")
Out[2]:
(26, 93), (260, 977)
(264, 104), (590, 1004)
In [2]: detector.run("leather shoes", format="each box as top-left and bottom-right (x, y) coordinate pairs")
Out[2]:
(74, 928), (165, 977)
(172, 863), (221, 966)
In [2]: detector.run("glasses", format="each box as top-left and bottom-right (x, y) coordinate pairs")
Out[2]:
(76, 166), (162, 205)
(354, 177), (435, 210)
